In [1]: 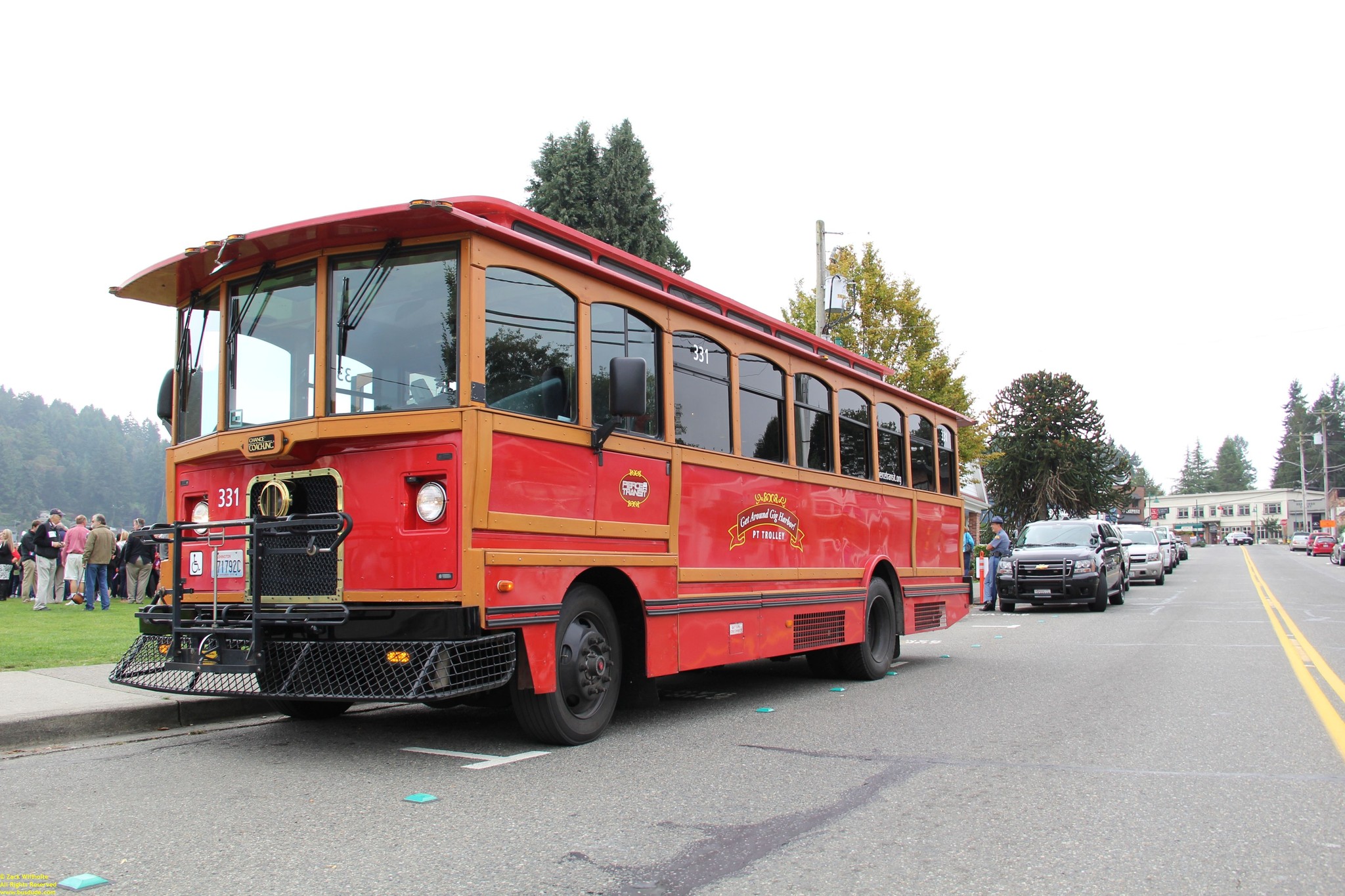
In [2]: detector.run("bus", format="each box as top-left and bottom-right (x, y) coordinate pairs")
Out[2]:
(106, 195), (980, 749)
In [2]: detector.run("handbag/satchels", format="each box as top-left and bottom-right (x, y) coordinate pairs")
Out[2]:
(965, 543), (972, 552)
(71, 593), (84, 604)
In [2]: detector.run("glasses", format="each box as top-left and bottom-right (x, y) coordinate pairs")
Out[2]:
(92, 521), (99, 523)
(136, 518), (140, 525)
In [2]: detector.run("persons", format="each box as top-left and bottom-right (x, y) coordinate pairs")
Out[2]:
(61, 515), (93, 605)
(107, 518), (169, 604)
(963, 526), (974, 576)
(33, 508), (66, 611)
(82, 513), (110, 611)
(0, 519), (71, 604)
(979, 516), (1009, 611)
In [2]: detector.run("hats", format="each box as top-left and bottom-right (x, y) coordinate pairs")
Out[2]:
(988, 516), (1004, 523)
(50, 508), (65, 516)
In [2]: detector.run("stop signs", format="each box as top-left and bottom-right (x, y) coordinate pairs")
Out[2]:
(1281, 519), (1287, 525)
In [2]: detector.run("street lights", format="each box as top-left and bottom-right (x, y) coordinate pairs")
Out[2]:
(1252, 504), (1258, 544)
(1148, 491), (1160, 526)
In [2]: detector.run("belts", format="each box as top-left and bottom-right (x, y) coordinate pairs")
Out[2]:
(989, 553), (1002, 557)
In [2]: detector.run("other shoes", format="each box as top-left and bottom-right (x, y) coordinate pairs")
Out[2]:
(96, 593), (102, 601)
(65, 600), (75, 605)
(979, 602), (996, 611)
(66, 595), (73, 599)
(82, 608), (87, 610)
(39, 607), (51, 611)
(15, 595), (18, 598)
(30, 597), (35, 600)
(9, 594), (15, 598)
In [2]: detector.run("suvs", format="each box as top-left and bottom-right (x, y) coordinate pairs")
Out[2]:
(995, 517), (1133, 613)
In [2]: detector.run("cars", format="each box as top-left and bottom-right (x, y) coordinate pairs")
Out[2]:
(1115, 524), (1187, 586)
(1288, 532), (1345, 566)
(1224, 532), (1253, 546)
